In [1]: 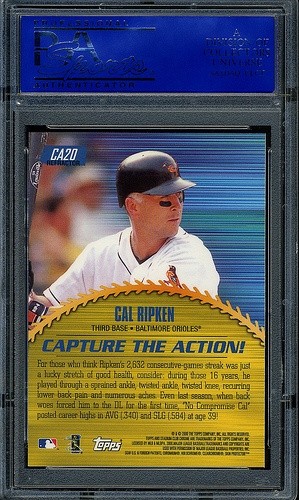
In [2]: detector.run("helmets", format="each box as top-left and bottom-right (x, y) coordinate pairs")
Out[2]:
(116, 151), (196, 208)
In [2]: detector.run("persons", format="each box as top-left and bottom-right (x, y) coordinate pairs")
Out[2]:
(27, 149), (222, 332)
